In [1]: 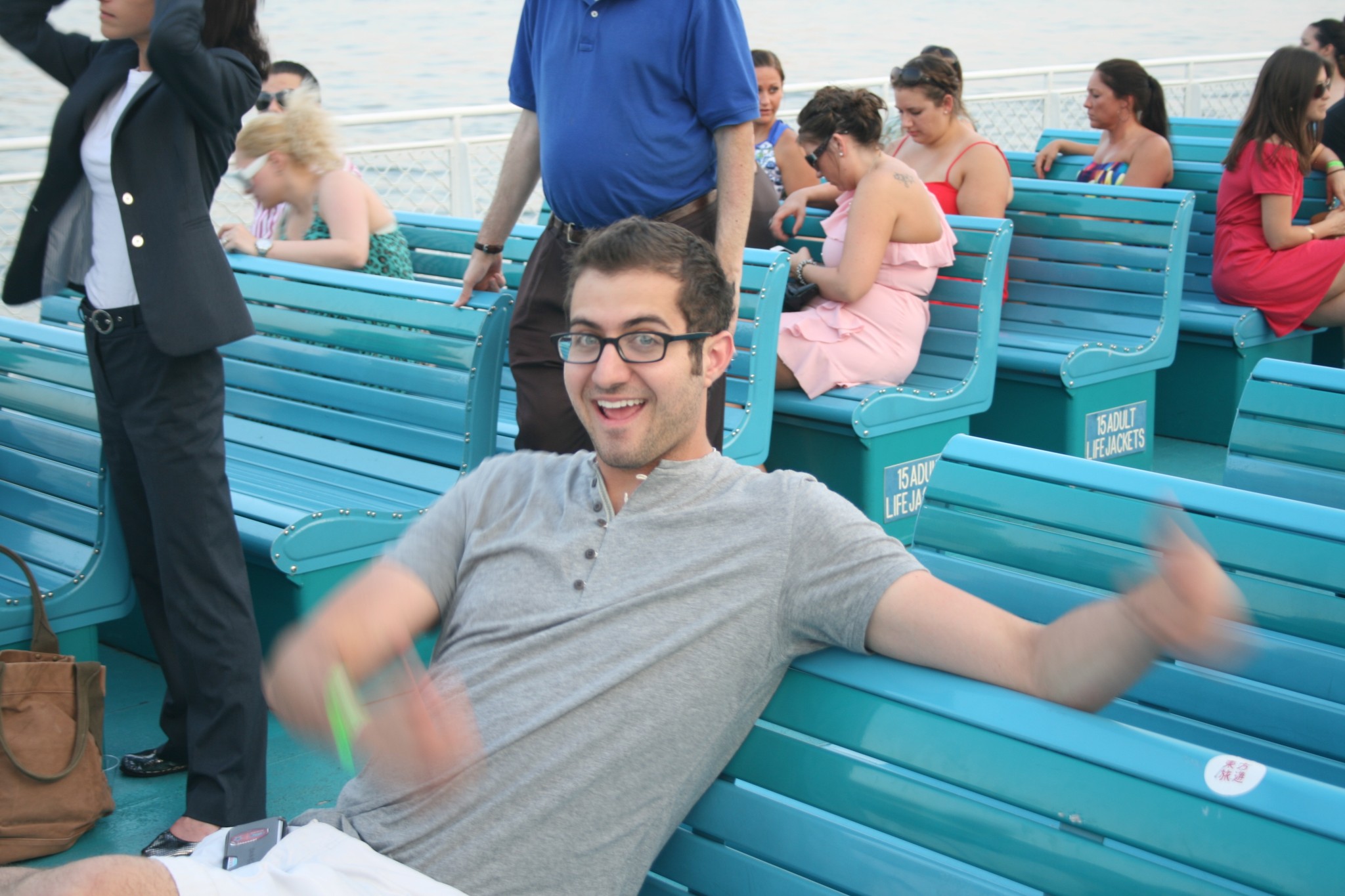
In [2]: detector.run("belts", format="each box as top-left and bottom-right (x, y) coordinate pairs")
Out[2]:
(77, 300), (143, 335)
(549, 188), (720, 247)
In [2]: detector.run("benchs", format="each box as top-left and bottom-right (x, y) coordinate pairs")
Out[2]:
(2, 116), (1345, 896)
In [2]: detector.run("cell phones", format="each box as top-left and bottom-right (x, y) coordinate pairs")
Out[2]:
(770, 245), (796, 255)
(224, 815), (285, 872)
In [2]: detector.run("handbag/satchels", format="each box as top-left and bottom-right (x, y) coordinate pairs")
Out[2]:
(0, 546), (117, 866)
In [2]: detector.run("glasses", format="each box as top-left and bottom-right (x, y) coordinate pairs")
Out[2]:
(922, 43), (955, 61)
(1313, 78), (1333, 100)
(232, 152), (268, 190)
(889, 66), (943, 90)
(254, 89), (294, 110)
(805, 128), (849, 172)
(549, 329), (712, 363)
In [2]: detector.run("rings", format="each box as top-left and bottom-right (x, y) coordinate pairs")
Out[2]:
(767, 218), (773, 224)
(221, 236), (229, 243)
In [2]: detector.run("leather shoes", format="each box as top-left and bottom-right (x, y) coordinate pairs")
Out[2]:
(123, 741), (186, 777)
(143, 828), (199, 859)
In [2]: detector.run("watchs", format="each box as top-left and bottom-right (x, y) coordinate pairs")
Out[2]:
(254, 236), (273, 258)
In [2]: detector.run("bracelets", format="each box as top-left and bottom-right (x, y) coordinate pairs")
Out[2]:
(474, 241), (504, 254)
(1305, 225), (1316, 240)
(1326, 168), (1345, 176)
(796, 259), (818, 285)
(324, 681), (372, 774)
(1326, 160), (1344, 169)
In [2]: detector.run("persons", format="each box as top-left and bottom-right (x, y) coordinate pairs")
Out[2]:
(1005, 58), (1176, 308)
(246, 57), (331, 259)
(0, 0), (275, 860)
(211, 75), (431, 395)
(879, 40), (1012, 311)
(749, 46), (824, 209)
(1209, 42), (1344, 335)
(0, 216), (1250, 896)
(451, 0), (766, 457)
(719, 83), (960, 473)
(1298, 16), (1345, 166)
